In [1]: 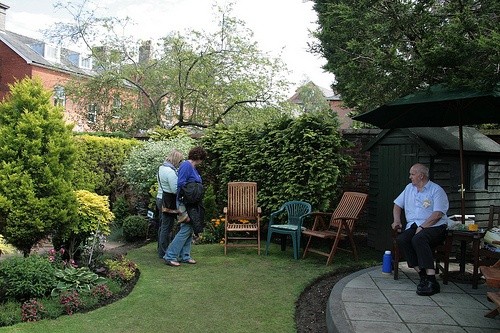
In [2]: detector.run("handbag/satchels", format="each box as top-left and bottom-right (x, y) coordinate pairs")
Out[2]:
(162, 191), (178, 214)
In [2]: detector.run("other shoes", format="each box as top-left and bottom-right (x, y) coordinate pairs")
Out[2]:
(186, 259), (197, 264)
(169, 261), (180, 266)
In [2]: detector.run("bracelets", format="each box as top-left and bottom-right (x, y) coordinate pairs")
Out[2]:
(418, 225), (424, 230)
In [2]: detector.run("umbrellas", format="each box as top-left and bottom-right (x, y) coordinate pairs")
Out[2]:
(351, 87), (500, 226)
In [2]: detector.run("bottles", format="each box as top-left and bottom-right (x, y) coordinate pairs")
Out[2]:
(382, 251), (392, 273)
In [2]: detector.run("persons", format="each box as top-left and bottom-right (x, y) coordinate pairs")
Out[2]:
(157, 150), (183, 258)
(163, 148), (206, 266)
(391, 163), (452, 295)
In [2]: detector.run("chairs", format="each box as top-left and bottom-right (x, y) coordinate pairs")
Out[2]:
(265, 201), (312, 263)
(223, 182), (263, 257)
(390, 205), (500, 320)
(301, 191), (368, 266)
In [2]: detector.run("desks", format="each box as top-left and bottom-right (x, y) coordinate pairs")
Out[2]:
(441, 227), (485, 290)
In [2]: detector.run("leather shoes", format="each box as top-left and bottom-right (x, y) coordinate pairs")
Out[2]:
(417, 275), (428, 290)
(416, 280), (440, 296)
